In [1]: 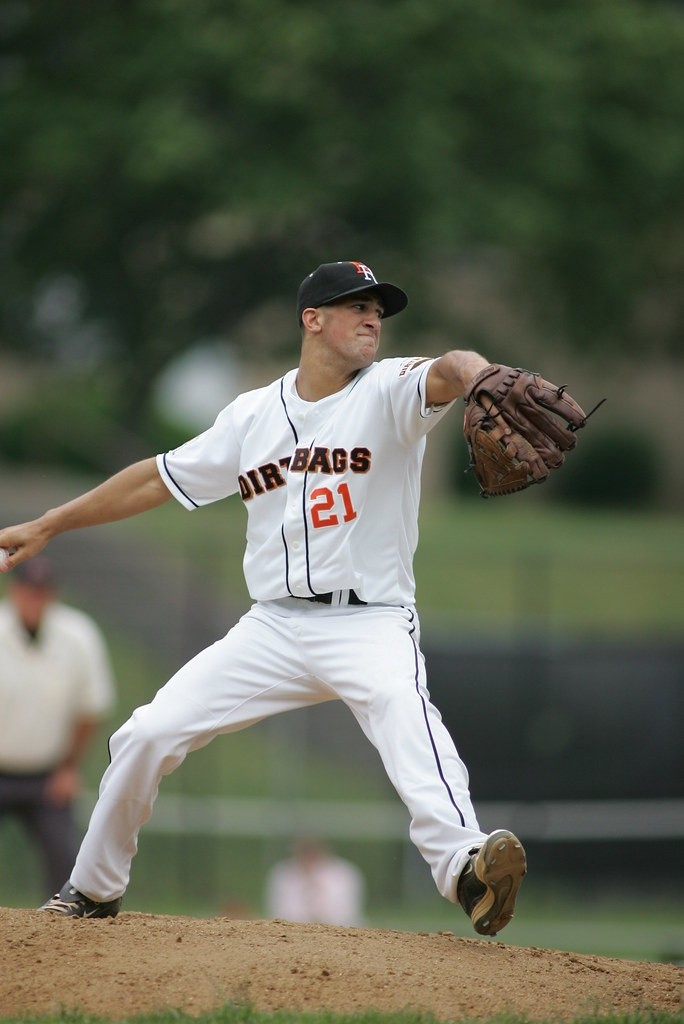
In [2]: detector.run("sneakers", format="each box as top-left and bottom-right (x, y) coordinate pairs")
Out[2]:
(37, 879), (122, 919)
(456, 828), (528, 935)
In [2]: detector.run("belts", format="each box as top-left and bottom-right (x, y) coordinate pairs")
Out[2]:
(286, 590), (364, 607)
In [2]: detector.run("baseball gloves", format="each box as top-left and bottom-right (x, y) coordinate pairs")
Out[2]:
(460, 359), (589, 500)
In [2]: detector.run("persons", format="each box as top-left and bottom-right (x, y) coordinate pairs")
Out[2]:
(0, 263), (526, 937)
(1, 556), (117, 905)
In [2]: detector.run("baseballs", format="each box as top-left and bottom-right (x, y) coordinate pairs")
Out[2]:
(0, 547), (10, 565)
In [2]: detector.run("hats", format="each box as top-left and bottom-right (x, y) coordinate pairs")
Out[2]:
(297, 261), (409, 319)
